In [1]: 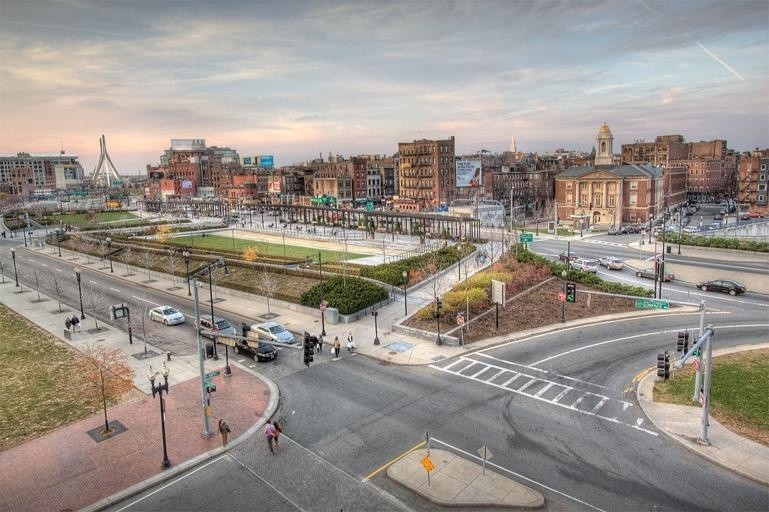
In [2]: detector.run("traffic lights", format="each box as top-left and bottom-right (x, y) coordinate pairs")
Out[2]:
(437, 300), (443, 309)
(658, 351), (669, 380)
(303, 332), (317, 367)
(677, 331), (689, 353)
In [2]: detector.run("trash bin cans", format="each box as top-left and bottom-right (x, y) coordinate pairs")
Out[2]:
(325, 308), (339, 324)
(206, 343), (213, 358)
(667, 246), (671, 253)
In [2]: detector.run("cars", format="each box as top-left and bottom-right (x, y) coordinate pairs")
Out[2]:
(229, 204), (278, 219)
(232, 337), (277, 362)
(252, 321), (294, 345)
(558, 252), (578, 263)
(696, 278), (745, 296)
(598, 256), (623, 270)
(636, 268), (674, 281)
(712, 204), (735, 230)
(149, 306), (186, 326)
(569, 259), (597, 275)
(608, 225), (648, 235)
(740, 211), (764, 220)
(195, 314), (235, 340)
(666, 206), (699, 233)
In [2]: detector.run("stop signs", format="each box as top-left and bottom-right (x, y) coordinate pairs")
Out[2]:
(320, 304), (326, 312)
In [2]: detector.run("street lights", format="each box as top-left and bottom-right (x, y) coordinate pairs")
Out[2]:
(148, 362), (170, 467)
(648, 213), (653, 244)
(678, 202), (682, 254)
(183, 251), (191, 296)
(9, 248), (19, 286)
(403, 270), (408, 316)
(74, 268), (85, 319)
(54, 227), (62, 259)
(562, 270), (567, 322)
(106, 237), (113, 272)
(654, 232), (659, 299)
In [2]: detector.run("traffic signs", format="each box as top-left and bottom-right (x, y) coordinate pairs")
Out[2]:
(366, 202), (374, 211)
(520, 234), (533, 242)
(422, 456), (435, 473)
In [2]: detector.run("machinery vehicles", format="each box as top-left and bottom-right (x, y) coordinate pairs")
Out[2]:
(310, 196), (336, 210)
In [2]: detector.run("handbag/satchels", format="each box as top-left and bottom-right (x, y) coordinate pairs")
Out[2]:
(331, 347), (336, 354)
(225, 424), (231, 433)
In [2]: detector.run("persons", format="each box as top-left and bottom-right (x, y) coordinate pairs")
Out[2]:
(318, 334), (324, 353)
(316, 343), (319, 352)
(218, 418), (230, 447)
(273, 421), (282, 449)
(264, 419), (277, 454)
(346, 330), (355, 351)
(332, 335), (341, 357)
(63, 316), (73, 330)
(71, 314), (81, 334)
